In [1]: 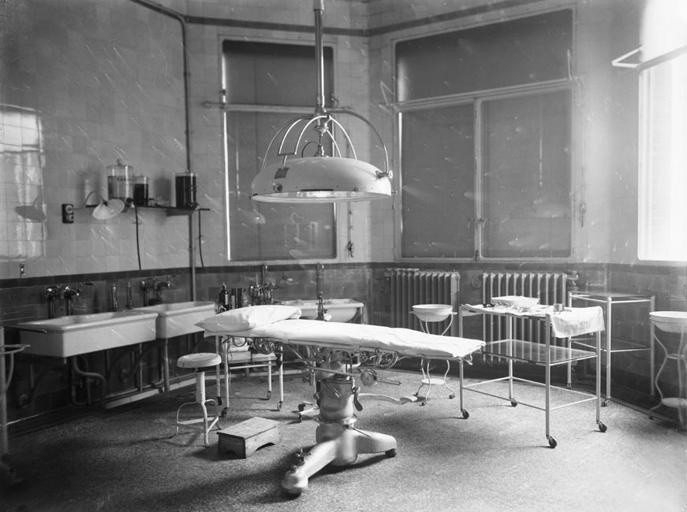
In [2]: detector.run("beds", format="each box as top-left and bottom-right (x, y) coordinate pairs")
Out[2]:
(203, 319), (485, 497)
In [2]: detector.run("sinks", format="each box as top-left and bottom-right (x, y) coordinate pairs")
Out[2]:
(279, 297), (364, 321)
(122, 300), (216, 339)
(5, 311), (159, 359)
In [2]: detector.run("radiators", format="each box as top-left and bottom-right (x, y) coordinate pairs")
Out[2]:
(385, 268), (576, 370)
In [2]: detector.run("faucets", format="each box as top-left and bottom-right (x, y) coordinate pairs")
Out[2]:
(64, 286), (80, 298)
(315, 264), (325, 300)
(154, 280), (170, 291)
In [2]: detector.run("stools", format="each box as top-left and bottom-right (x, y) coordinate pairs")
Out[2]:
(174, 353), (221, 448)
(217, 416), (279, 457)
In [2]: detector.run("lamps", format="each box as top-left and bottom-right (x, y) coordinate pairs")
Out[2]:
(248, 1), (394, 201)
